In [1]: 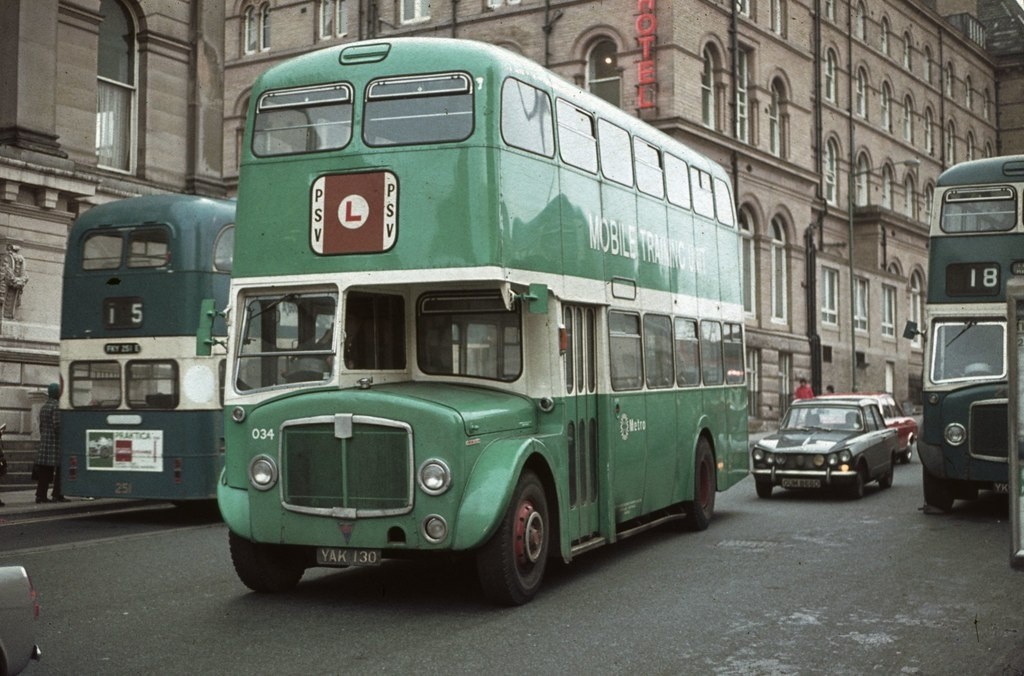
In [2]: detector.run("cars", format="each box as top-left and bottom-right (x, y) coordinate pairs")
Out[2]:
(753, 395), (901, 500)
(0, 566), (41, 676)
(820, 390), (918, 464)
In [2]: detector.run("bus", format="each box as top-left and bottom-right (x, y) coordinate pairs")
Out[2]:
(216, 33), (753, 593)
(56, 192), (242, 523)
(916, 153), (1024, 516)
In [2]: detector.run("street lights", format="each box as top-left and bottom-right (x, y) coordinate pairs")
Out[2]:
(848, 158), (922, 393)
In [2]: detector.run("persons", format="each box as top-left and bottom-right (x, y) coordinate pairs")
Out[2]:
(827, 385), (834, 394)
(796, 379), (813, 399)
(35, 383), (71, 504)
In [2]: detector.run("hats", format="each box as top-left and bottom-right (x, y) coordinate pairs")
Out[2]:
(48, 383), (61, 395)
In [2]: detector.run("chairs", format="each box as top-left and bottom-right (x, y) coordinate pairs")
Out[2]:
(844, 411), (861, 430)
(803, 414), (822, 427)
(319, 328), (354, 371)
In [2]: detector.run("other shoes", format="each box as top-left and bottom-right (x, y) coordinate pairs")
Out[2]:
(51, 495), (71, 503)
(35, 496), (51, 503)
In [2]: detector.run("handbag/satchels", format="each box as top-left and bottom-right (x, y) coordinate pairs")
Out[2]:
(31, 463), (55, 483)
